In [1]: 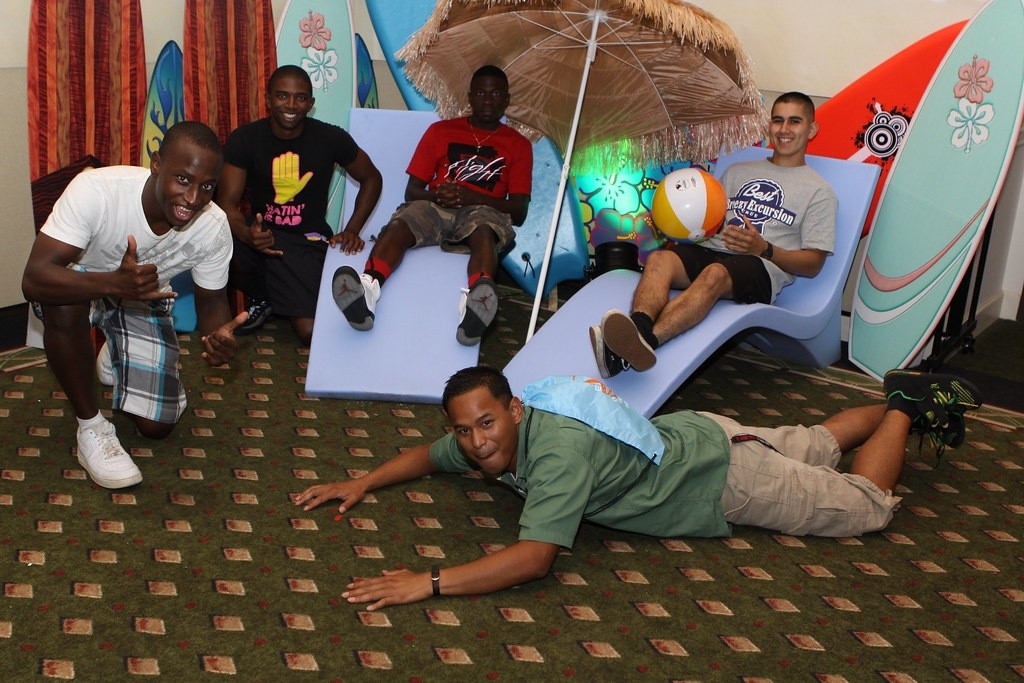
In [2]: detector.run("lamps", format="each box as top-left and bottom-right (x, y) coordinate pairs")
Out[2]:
(584, 242), (645, 281)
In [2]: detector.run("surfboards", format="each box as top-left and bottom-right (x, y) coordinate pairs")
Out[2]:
(710, 19), (970, 240)
(275, 0), (357, 236)
(365, 0), (591, 300)
(354, 33), (379, 109)
(139, 39), (198, 333)
(848, 0), (1024, 383)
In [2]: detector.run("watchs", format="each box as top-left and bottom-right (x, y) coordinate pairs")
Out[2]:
(760, 241), (773, 260)
(431, 566), (439, 600)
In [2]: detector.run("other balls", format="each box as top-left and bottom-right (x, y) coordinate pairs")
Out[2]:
(652, 169), (728, 244)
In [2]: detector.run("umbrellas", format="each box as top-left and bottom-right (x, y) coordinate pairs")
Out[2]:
(392, 0), (766, 345)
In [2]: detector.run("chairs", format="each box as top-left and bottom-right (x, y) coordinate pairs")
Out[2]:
(500, 140), (882, 420)
(303, 107), (483, 403)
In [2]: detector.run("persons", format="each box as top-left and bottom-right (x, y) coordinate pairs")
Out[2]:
(332, 65), (533, 347)
(21, 121), (247, 488)
(296, 366), (983, 611)
(212, 65), (383, 344)
(589, 91), (839, 378)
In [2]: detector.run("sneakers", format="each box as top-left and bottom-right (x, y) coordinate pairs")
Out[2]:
(589, 325), (631, 379)
(877, 373), (984, 469)
(332, 266), (381, 332)
(235, 297), (272, 331)
(600, 309), (656, 372)
(77, 420), (143, 489)
(456, 276), (499, 346)
(97, 341), (114, 386)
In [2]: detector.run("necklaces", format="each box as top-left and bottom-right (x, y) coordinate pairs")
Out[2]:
(469, 119), (500, 152)
